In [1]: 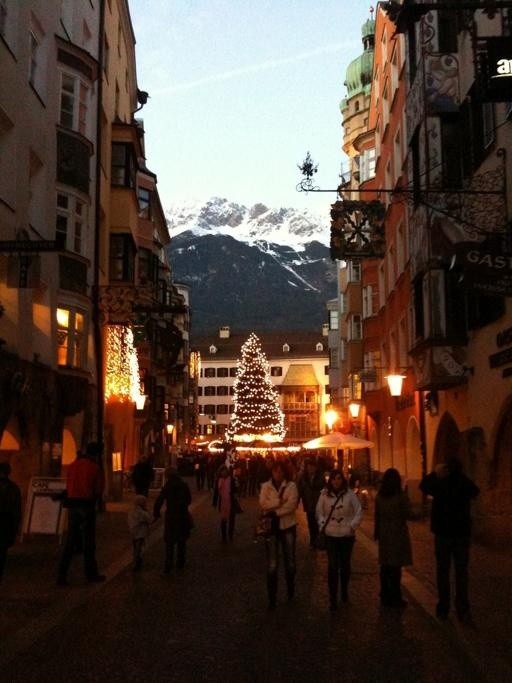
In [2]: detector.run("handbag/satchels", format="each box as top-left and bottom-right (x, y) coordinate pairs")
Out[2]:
(230, 498), (244, 515)
(314, 532), (329, 551)
(252, 520), (276, 545)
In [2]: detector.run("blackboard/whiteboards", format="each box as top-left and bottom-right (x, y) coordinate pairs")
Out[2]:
(22, 477), (67, 535)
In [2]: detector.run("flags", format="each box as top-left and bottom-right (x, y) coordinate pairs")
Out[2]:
(6, 254), (41, 289)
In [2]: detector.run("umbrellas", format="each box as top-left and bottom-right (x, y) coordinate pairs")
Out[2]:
(303, 430), (376, 450)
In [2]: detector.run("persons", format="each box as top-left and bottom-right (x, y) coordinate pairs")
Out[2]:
(371, 467), (423, 610)
(131, 454), (156, 497)
(418, 463), (480, 621)
(56, 440), (107, 586)
(178, 448), (360, 498)
(153, 468), (192, 576)
(211, 466), (238, 544)
(258, 460), (299, 611)
(297, 460), (326, 550)
(315, 469), (363, 602)
(127, 495), (150, 563)
(0, 461), (22, 579)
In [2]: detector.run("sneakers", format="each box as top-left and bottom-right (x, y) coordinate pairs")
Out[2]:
(328, 593), (349, 610)
(83, 572), (106, 582)
(56, 573), (72, 586)
(378, 595), (409, 608)
(435, 601), (472, 622)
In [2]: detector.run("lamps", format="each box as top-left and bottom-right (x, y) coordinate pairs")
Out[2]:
(322, 366), (420, 430)
